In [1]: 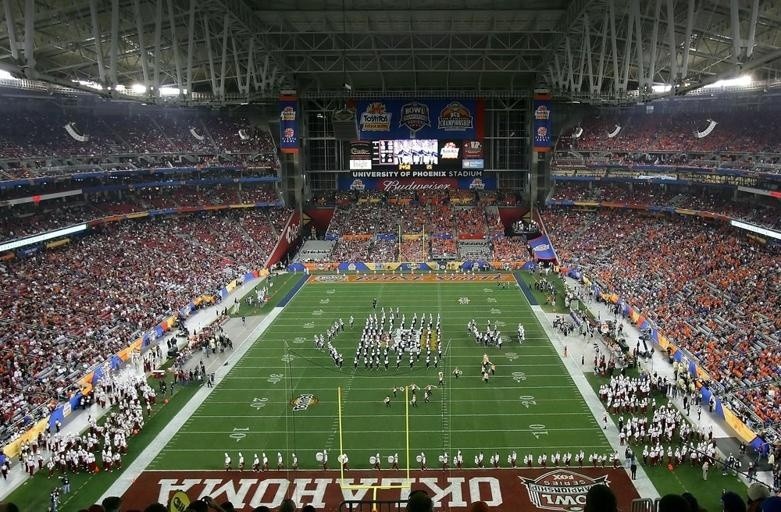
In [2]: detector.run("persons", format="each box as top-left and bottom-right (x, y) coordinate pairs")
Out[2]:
(0, 107), (781, 511)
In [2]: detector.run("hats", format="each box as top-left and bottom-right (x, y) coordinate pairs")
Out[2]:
(19, 362), (154, 461)
(354, 306), (442, 361)
(600, 374), (714, 453)
(224, 451), (462, 458)
(314, 316), (353, 358)
(467, 319), (527, 340)
